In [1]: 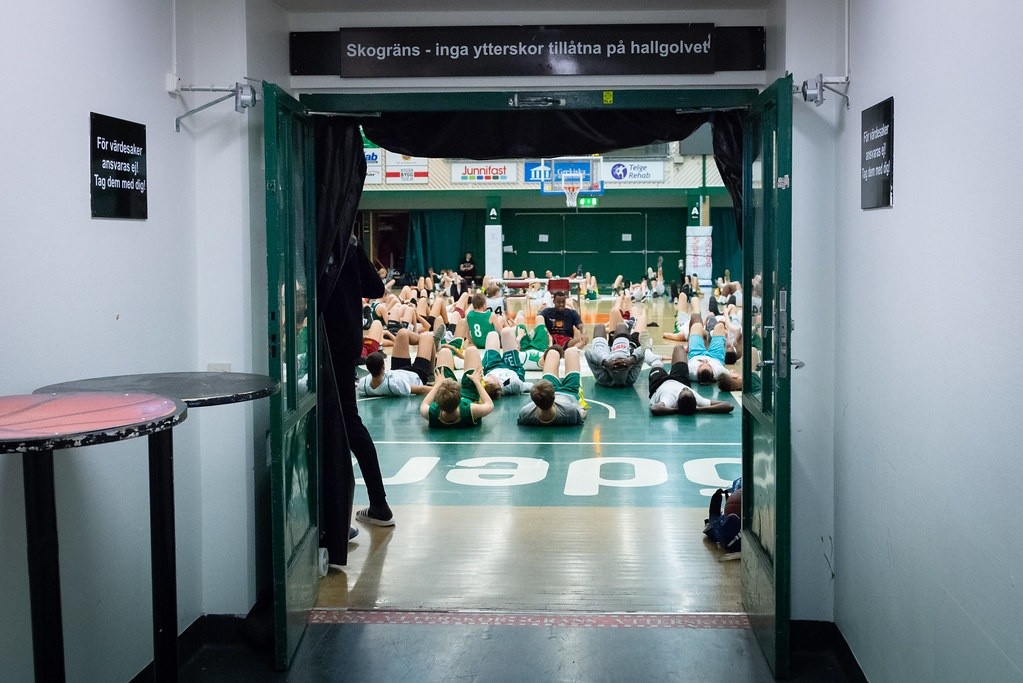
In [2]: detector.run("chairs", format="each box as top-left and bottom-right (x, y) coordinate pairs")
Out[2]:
(547, 279), (570, 293)
(508, 280), (529, 296)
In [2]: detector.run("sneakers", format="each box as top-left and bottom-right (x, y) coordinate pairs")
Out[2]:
(625, 317), (636, 331)
(638, 329), (653, 355)
(427, 375), (435, 386)
(433, 324), (446, 352)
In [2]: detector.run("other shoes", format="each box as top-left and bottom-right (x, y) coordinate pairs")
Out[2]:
(718, 277), (723, 285)
(648, 268), (653, 279)
(653, 292), (657, 298)
(631, 296), (635, 303)
(724, 270), (729, 281)
(641, 296), (648, 303)
(657, 256), (663, 268)
(692, 292), (698, 297)
(674, 299), (677, 304)
(678, 260), (683, 268)
(682, 283), (689, 301)
(379, 350), (387, 359)
(364, 305), (371, 319)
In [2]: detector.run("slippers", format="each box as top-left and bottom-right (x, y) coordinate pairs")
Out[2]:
(355, 509), (395, 527)
(348, 526), (359, 540)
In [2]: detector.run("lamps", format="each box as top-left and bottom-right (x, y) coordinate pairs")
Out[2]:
(165, 73), (257, 133)
(801, 74), (852, 112)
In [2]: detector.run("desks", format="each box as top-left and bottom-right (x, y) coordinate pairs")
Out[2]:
(0, 393), (188, 683)
(490, 277), (587, 293)
(32, 371), (281, 683)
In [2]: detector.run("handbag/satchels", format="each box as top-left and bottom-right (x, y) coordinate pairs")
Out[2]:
(703, 477), (742, 553)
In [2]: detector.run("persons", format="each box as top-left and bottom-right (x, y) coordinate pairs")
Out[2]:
(459, 252), (476, 284)
(296, 308), (307, 380)
(540, 292), (589, 347)
(359, 256), (762, 426)
(324, 235), (394, 539)
